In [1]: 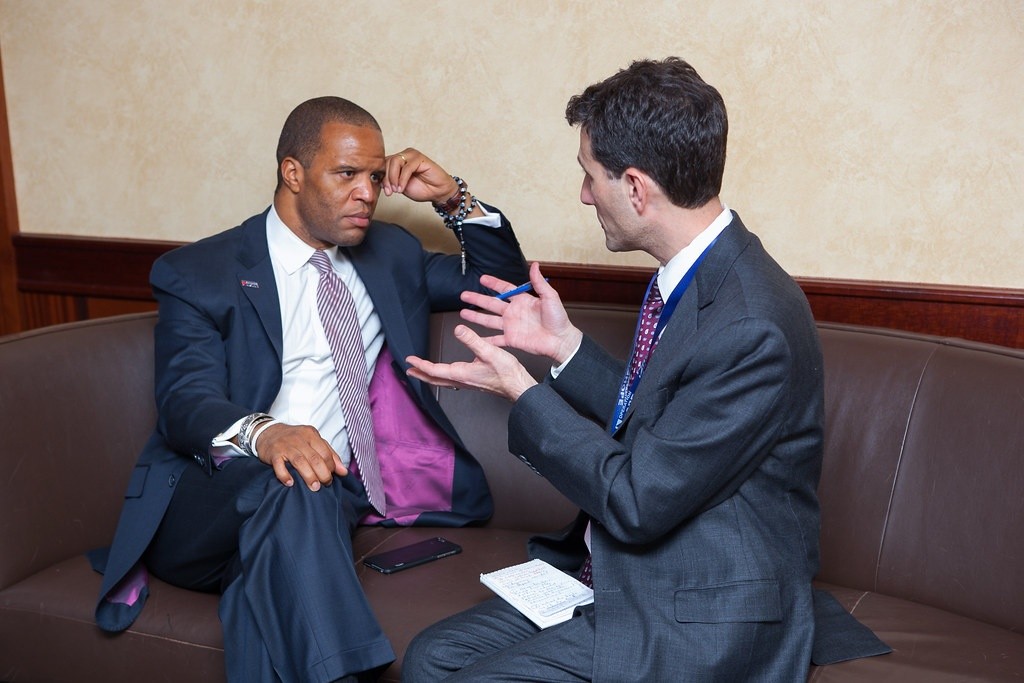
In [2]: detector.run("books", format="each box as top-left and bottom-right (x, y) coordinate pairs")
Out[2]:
(480, 559), (594, 630)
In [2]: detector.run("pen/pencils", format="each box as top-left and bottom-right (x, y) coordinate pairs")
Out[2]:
(495, 277), (548, 301)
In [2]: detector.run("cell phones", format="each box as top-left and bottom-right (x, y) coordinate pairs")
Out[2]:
(362, 536), (463, 574)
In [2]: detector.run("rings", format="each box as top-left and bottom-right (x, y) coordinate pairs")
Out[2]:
(453, 387), (459, 391)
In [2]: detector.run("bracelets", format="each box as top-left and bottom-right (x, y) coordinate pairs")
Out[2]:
(238, 412), (287, 457)
(433, 175), (477, 276)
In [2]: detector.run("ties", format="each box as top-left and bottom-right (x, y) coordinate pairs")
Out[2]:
(579, 275), (664, 588)
(308, 250), (386, 517)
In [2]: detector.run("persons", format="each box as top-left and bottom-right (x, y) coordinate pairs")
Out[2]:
(83, 96), (535, 682)
(403, 54), (893, 683)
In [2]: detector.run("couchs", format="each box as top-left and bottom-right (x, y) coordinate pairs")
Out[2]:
(0, 302), (1024, 683)
